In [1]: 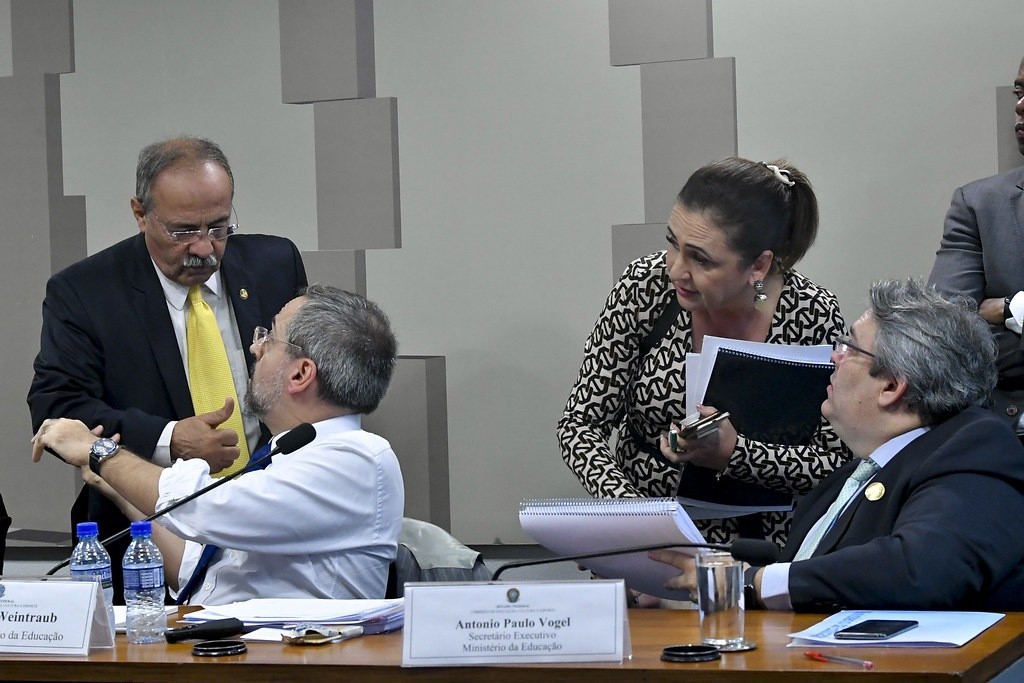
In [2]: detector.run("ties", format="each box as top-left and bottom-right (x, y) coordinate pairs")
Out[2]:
(176, 442), (271, 605)
(186, 284), (250, 480)
(791, 459), (881, 562)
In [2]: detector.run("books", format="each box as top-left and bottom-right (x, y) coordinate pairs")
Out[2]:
(175, 596), (405, 637)
(517, 497), (713, 602)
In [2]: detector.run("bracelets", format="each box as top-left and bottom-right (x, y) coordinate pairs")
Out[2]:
(1003, 289), (1024, 331)
(743, 560), (765, 608)
(716, 460), (729, 482)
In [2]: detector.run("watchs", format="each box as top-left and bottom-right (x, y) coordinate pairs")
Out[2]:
(88, 437), (127, 477)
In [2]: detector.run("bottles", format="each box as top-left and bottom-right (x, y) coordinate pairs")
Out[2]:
(69, 522), (115, 639)
(122, 521), (167, 643)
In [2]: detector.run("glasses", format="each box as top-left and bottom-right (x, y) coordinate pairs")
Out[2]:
(253, 326), (303, 350)
(833, 335), (876, 359)
(150, 204), (239, 244)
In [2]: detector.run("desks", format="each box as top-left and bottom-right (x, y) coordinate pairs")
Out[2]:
(0, 608), (1024, 683)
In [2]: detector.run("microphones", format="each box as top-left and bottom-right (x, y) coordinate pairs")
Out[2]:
(46, 423), (317, 576)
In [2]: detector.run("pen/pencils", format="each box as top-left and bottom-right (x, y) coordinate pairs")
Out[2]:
(669, 429), (679, 453)
(804, 651), (873, 669)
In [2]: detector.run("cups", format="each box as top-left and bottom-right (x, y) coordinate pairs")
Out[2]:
(695, 552), (745, 643)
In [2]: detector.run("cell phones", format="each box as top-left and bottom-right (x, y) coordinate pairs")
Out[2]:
(835, 620), (919, 640)
(678, 411), (731, 438)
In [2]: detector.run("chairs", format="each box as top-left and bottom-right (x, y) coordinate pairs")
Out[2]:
(394, 517), (502, 599)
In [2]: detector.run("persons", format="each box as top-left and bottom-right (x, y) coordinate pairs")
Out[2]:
(925, 51), (1024, 447)
(554, 156), (853, 613)
(26, 134), (310, 608)
(29, 282), (406, 606)
(644, 274), (1024, 613)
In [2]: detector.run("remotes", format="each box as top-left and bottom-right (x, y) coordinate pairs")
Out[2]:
(163, 618), (243, 644)
